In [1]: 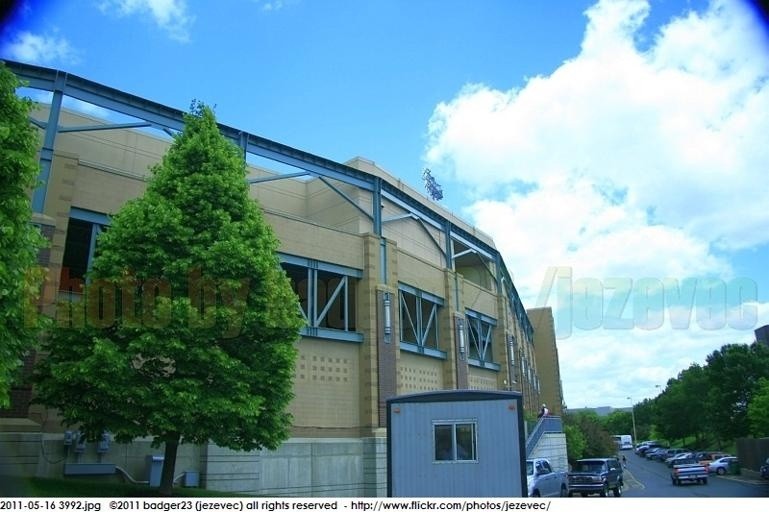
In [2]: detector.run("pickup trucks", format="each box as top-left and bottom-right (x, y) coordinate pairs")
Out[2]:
(526, 458), (569, 497)
(670, 458), (708, 486)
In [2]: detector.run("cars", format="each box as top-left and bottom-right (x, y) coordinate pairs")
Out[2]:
(634, 441), (738, 476)
(760, 458), (769, 481)
(569, 458), (624, 497)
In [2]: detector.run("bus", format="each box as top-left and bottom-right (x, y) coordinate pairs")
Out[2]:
(609, 435), (633, 450)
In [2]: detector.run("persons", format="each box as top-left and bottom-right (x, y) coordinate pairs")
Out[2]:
(536, 402), (549, 418)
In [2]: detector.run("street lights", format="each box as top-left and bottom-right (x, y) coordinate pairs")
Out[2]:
(626, 397), (637, 443)
(656, 385), (662, 393)
